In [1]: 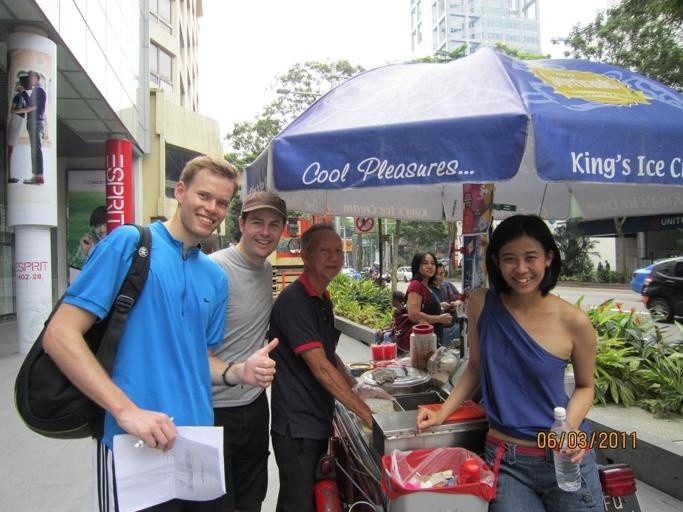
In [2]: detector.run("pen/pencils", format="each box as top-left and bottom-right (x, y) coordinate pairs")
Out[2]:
(132, 417), (174, 450)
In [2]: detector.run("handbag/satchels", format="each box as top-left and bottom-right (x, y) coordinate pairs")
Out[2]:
(14, 224), (152, 441)
(389, 279), (426, 352)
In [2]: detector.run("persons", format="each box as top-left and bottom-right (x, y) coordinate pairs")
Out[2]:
(417, 215), (606, 512)
(597, 262), (603, 272)
(404, 252), (453, 350)
(605, 260), (610, 272)
(268, 225), (396, 510)
(8, 70), (45, 186)
(205, 192), (288, 512)
(73, 205), (108, 261)
(40, 156), (279, 512)
(429, 261), (463, 350)
(374, 292), (412, 352)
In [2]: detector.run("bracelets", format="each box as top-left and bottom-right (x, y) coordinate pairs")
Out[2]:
(222, 362), (238, 390)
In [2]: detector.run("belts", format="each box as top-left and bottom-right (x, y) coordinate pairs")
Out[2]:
(486, 434), (594, 456)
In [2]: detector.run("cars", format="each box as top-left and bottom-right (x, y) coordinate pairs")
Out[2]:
(630, 258), (683, 293)
(641, 258), (683, 322)
(397, 267), (413, 282)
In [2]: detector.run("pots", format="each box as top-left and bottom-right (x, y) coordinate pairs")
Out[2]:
(360, 364), (433, 396)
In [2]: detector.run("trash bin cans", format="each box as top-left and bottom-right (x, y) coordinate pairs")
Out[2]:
(379, 447), (495, 512)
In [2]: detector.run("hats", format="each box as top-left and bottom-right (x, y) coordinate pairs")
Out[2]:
(241, 190), (287, 218)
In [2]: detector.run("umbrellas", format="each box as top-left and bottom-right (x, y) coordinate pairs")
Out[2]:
(242, 46), (682, 224)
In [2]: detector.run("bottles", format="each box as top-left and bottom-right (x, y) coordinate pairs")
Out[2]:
(409, 323), (437, 371)
(551, 406), (582, 492)
